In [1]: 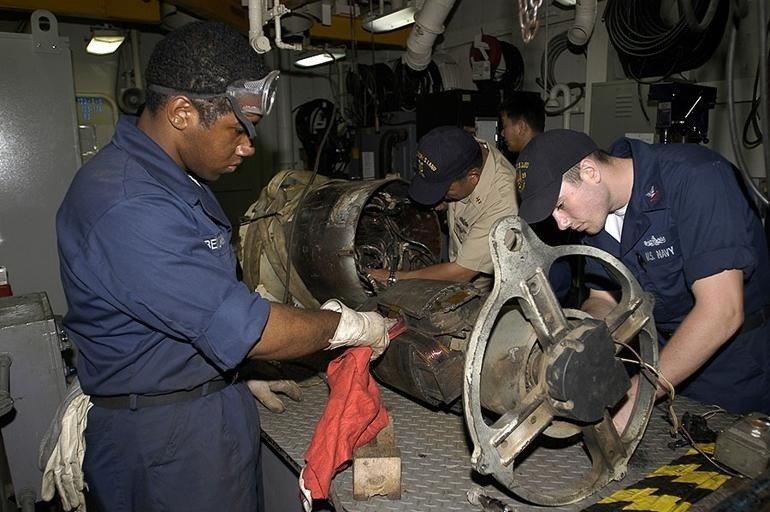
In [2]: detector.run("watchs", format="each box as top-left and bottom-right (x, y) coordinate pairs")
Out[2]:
(386, 271), (397, 287)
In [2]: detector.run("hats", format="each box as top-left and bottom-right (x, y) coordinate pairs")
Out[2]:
(406, 125), (479, 206)
(515, 129), (598, 224)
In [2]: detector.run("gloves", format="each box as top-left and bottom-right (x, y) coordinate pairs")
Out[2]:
(319, 299), (397, 360)
(238, 358), (304, 411)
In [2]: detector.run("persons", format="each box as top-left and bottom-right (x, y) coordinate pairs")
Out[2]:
(499, 91), (546, 155)
(361, 124), (522, 287)
(55, 20), (399, 512)
(516, 125), (770, 416)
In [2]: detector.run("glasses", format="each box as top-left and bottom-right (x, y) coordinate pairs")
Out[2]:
(225, 69), (280, 118)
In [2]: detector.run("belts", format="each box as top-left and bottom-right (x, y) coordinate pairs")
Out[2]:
(87, 378), (230, 410)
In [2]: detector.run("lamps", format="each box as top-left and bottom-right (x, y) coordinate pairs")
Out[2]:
(84, 3), (127, 55)
